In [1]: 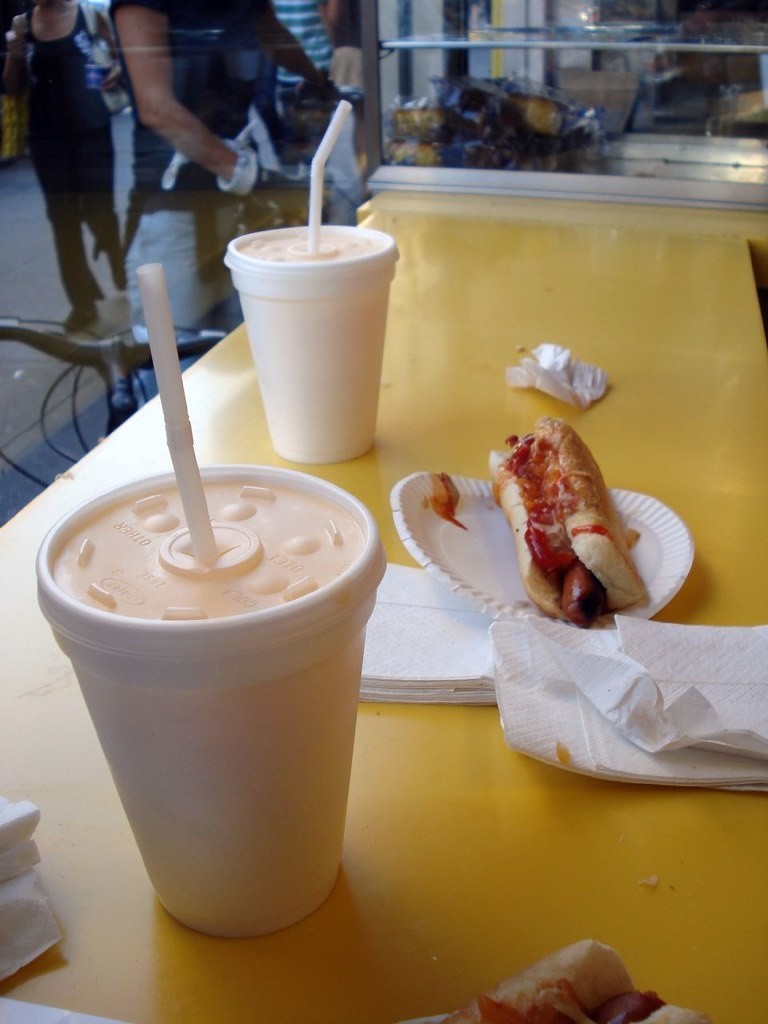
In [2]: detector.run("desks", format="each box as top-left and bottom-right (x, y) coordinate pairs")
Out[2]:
(0, 209), (768, 1024)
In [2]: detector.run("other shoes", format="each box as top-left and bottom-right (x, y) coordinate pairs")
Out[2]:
(108, 248), (127, 290)
(63, 301), (98, 334)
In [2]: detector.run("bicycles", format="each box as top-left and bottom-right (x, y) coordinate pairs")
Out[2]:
(0, 315), (227, 491)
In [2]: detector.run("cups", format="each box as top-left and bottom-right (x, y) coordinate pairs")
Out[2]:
(224, 225), (399, 465)
(35, 464), (386, 937)
(84, 64), (107, 90)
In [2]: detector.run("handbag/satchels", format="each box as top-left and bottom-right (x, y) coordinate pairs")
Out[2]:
(81, 3), (128, 113)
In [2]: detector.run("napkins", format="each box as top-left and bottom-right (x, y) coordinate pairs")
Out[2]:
(487, 614), (768, 791)
(357, 563), (500, 705)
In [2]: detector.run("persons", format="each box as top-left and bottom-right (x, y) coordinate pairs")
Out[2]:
(3, 0), (127, 331)
(108, 0), (284, 190)
(234, 0), (364, 116)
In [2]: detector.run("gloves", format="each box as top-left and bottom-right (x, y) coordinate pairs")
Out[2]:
(216, 138), (281, 196)
(294, 69), (340, 109)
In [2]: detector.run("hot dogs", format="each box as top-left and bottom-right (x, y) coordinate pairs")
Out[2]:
(433, 937), (717, 1024)
(488, 417), (646, 625)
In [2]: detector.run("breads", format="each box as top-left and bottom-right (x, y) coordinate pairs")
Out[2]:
(387, 92), (605, 174)
